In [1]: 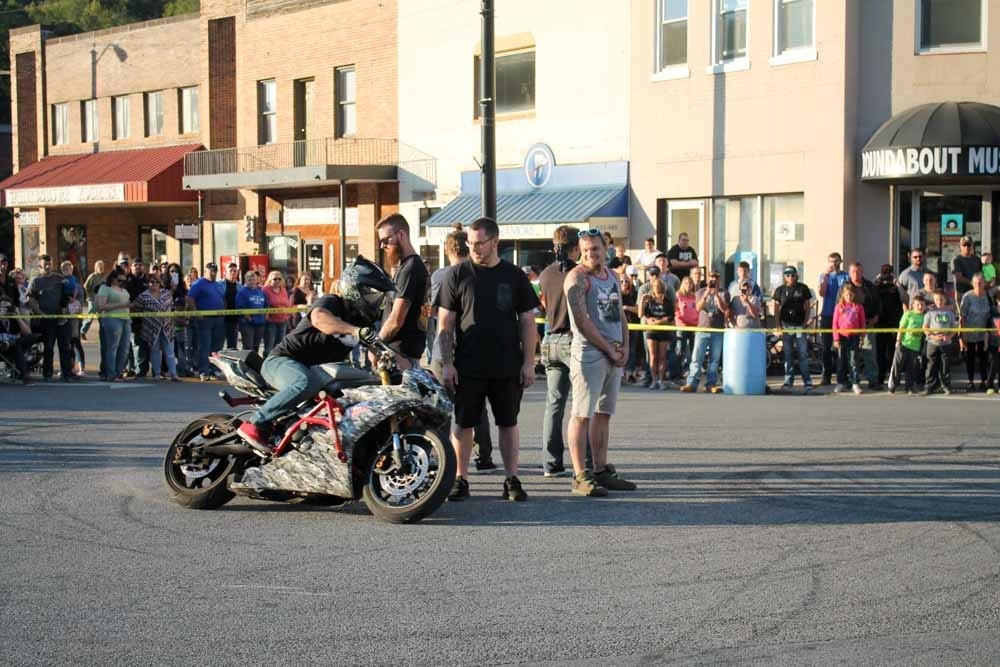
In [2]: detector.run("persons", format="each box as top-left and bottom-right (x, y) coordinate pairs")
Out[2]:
(376, 213), (431, 367)
(80, 260), (105, 340)
(772, 265), (813, 389)
(426, 231), (497, 470)
(604, 232), (763, 393)
(237, 254), (398, 454)
(431, 217), (541, 502)
(95, 250), (316, 381)
(520, 265), (542, 318)
(0, 253), (86, 385)
(538, 225), (615, 477)
(329, 278), (360, 369)
(563, 228), (636, 497)
(818, 236), (1000, 396)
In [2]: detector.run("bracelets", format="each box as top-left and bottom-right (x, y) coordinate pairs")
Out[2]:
(896, 340), (900, 342)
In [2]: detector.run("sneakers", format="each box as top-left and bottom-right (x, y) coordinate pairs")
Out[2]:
(235, 421), (271, 454)
(474, 461), (497, 473)
(571, 461), (636, 497)
(502, 475), (528, 501)
(447, 474), (471, 502)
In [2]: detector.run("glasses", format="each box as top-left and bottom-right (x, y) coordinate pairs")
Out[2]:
(116, 277), (126, 283)
(150, 270), (281, 285)
(630, 274), (638, 278)
(465, 235), (496, 249)
(913, 255), (923, 259)
(576, 227), (605, 245)
(378, 229), (401, 246)
(619, 279), (629, 283)
(649, 272), (659, 276)
(657, 291), (665, 294)
(963, 242), (972, 248)
(680, 238), (689, 242)
(786, 273), (797, 278)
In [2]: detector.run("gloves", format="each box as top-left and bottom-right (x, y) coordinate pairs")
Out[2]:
(356, 326), (376, 347)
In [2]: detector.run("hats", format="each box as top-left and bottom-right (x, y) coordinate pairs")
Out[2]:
(118, 256), (144, 267)
(625, 265), (798, 288)
(205, 262), (217, 270)
(960, 235), (973, 246)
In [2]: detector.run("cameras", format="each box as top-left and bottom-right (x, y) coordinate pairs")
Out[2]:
(742, 289), (747, 295)
(710, 280), (716, 288)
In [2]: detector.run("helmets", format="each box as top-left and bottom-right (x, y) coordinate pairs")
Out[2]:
(337, 254), (400, 326)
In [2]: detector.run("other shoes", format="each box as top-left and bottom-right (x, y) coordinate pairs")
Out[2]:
(620, 364), (722, 394)
(766, 379), (1000, 398)
(42, 368), (220, 383)
(543, 468), (573, 477)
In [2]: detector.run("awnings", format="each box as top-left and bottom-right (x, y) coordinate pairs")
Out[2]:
(421, 185), (628, 245)
(860, 101), (1000, 181)
(0, 143), (202, 218)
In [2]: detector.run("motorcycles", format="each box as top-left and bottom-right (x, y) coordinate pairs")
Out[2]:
(164, 327), (457, 524)
